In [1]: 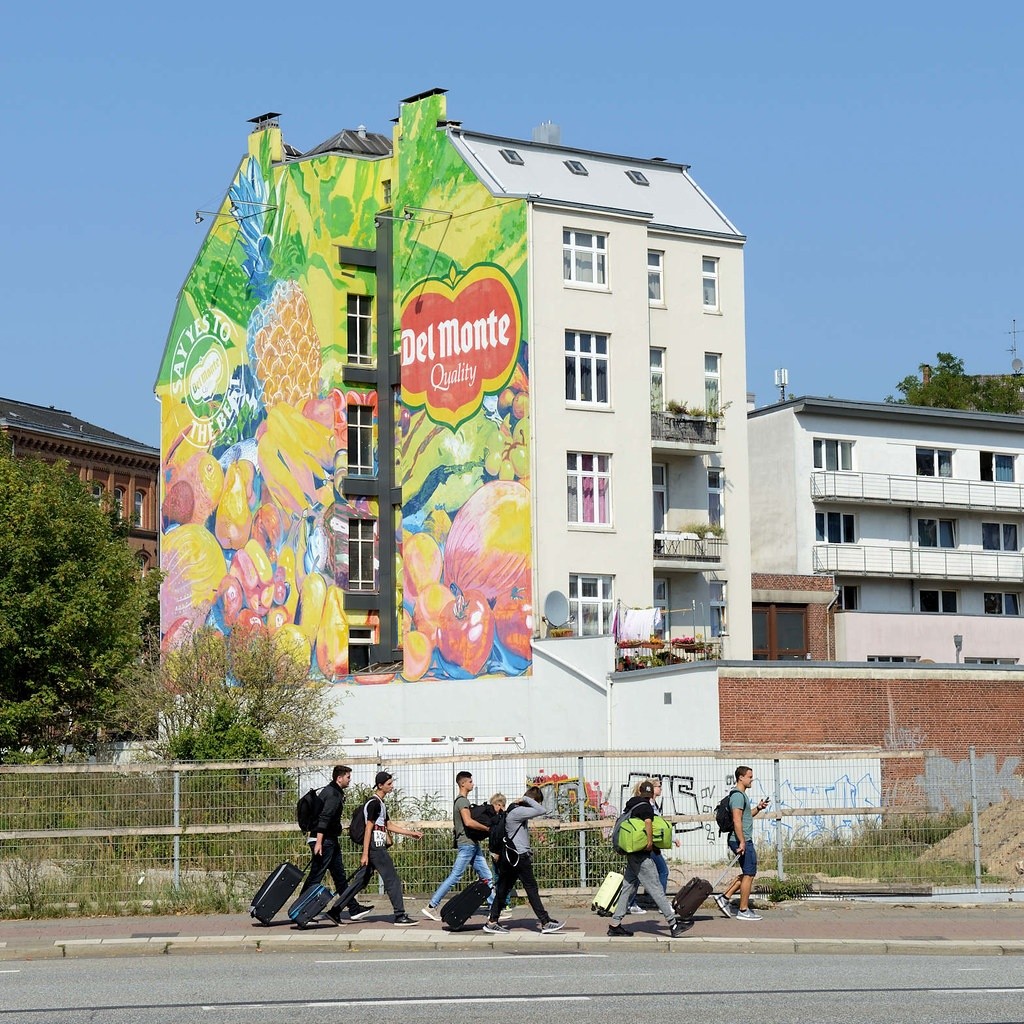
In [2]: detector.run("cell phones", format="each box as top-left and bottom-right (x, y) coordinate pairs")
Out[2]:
(761, 797), (769, 806)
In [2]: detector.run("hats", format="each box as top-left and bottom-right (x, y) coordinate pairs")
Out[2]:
(371, 772), (391, 790)
(640, 782), (654, 798)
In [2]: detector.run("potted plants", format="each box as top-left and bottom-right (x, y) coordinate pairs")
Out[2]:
(550, 628), (573, 638)
(678, 521), (725, 540)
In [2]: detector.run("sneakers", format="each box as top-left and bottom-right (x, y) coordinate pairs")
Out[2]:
(489, 909), (513, 921)
(393, 914), (420, 926)
(422, 904), (442, 921)
(669, 920), (694, 937)
(482, 920), (511, 934)
(713, 893), (763, 921)
(542, 919), (566, 934)
(627, 904), (647, 915)
(324, 910), (348, 926)
(350, 905), (376, 920)
(606, 924), (634, 936)
(659, 901), (676, 913)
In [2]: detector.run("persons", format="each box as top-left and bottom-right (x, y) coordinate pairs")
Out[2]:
(483, 786), (566, 934)
(607, 782), (694, 937)
(300, 766), (374, 919)
(488, 793), (513, 912)
(325, 771), (423, 927)
(627, 779), (675, 914)
(714, 766), (768, 920)
(422, 771), (512, 921)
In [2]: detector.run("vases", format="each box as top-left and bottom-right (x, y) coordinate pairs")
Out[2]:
(619, 644), (640, 648)
(642, 643), (665, 649)
(684, 647), (712, 653)
(673, 644), (695, 648)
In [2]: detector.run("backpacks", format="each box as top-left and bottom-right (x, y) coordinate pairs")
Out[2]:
(297, 784), (328, 838)
(611, 802), (648, 855)
(714, 790), (746, 845)
(349, 797), (375, 847)
(488, 801), (533, 870)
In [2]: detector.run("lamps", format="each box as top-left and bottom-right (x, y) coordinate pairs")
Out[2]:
(194, 210), (244, 224)
(403, 205), (453, 220)
(806, 651), (811, 659)
(373, 214), (422, 229)
(228, 200), (278, 213)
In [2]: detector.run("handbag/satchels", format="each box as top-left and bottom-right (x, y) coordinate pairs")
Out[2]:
(618, 815), (672, 853)
(462, 802), (499, 843)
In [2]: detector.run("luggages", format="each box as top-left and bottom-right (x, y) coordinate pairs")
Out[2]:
(440, 878), (499, 932)
(250, 851), (320, 927)
(287, 864), (365, 930)
(591, 871), (627, 916)
(671, 850), (743, 924)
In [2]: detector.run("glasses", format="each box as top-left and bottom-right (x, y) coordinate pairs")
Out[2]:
(654, 784), (661, 788)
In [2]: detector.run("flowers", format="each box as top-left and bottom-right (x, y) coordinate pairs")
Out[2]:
(671, 637), (695, 645)
(695, 634), (713, 648)
(617, 639), (641, 647)
(642, 638), (663, 644)
